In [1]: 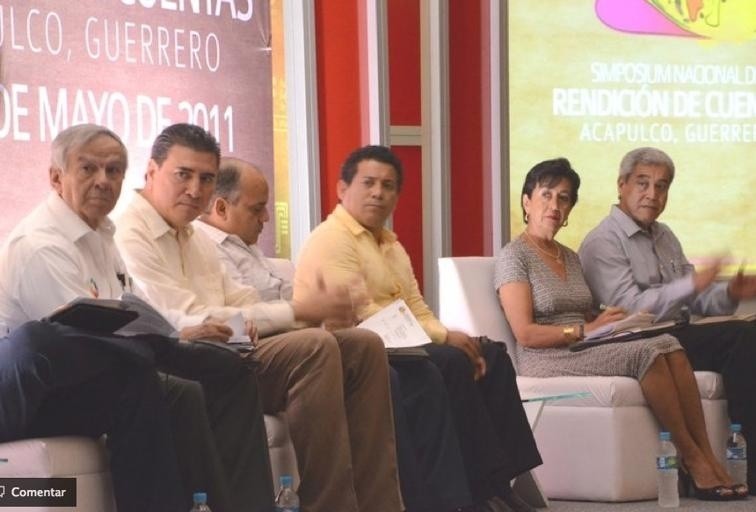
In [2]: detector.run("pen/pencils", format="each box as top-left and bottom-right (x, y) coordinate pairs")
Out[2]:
(597, 305), (616, 311)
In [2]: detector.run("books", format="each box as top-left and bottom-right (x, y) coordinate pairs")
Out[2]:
(347, 295), (432, 359)
(570, 313), (682, 355)
(41, 289), (183, 342)
(202, 312), (259, 354)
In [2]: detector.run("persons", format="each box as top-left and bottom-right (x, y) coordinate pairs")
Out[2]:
(0, 296), (190, 511)
(0, 123), (279, 512)
(513, 156), (749, 499)
(114, 123), (406, 512)
(576, 149), (756, 494)
(194, 158), (463, 512)
(293, 146), (534, 512)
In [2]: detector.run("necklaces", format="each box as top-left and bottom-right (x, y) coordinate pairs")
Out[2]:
(522, 225), (569, 269)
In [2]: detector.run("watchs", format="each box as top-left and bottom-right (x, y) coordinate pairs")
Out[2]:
(576, 322), (588, 341)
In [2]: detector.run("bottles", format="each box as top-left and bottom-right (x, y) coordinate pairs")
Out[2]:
(651, 431), (683, 508)
(191, 491), (212, 512)
(723, 424), (748, 491)
(275, 474), (301, 512)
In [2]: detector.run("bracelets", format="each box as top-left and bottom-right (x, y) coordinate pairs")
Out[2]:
(561, 322), (573, 340)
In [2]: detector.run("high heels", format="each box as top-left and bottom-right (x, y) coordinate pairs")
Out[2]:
(682, 462), (733, 500)
(716, 480), (749, 499)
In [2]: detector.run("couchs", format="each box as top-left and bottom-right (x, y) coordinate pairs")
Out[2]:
(0, 414), (299, 511)
(437, 256), (727, 501)
